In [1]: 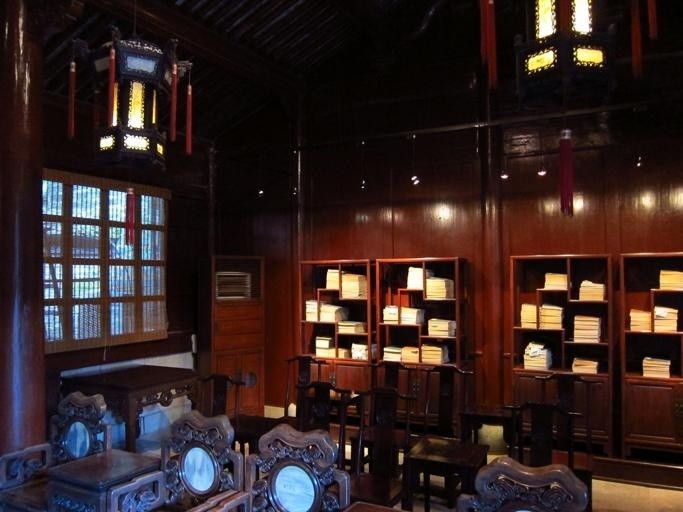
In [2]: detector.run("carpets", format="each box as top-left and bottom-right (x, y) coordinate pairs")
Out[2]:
(333, 442), (683, 512)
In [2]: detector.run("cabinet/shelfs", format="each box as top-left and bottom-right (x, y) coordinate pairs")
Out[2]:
(376, 257), (472, 433)
(617, 253), (682, 459)
(299, 259), (373, 428)
(507, 254), (614, 460)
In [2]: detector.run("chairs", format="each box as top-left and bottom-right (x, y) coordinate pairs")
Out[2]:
(1, 390), (589, 509)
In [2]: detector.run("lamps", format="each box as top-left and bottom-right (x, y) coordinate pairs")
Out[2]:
(518, 0), (612, 110)
(60, 0), (195, 177)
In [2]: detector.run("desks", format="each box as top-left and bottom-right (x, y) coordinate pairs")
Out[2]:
(402, 436), (490, 510)
(303, 390), (362, 444)
(46, 447), (162, 512)
(459, 405), (523, 449)
(57, 365), (198, 453)
(230, 414), (289, 468)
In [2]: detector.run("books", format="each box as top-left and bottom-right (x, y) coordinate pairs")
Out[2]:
(519, 263), (682, 378)
(305, 265), (456, 366)
